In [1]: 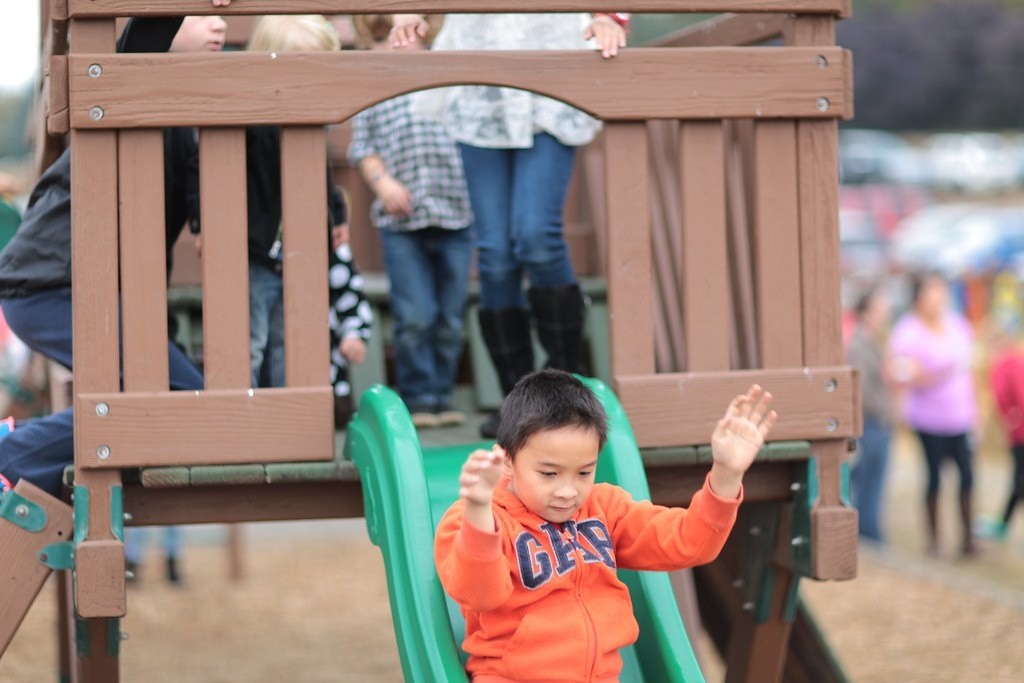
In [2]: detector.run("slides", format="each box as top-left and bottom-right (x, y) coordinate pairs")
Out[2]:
(343, 372), (707, 683)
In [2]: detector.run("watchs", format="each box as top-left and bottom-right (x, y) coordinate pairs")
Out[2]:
(613, 13), (630, 26)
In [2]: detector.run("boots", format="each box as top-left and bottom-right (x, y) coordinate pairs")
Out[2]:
(527, 279), (595, 379)
(479, 305), (532, 438)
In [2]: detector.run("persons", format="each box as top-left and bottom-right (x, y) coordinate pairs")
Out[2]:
(0, 0), (627, 499)
(849, 270), (1024, 560)
(434, 368), (778, 683)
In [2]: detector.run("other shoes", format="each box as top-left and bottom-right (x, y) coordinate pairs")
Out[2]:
(414, 411), (464, 428)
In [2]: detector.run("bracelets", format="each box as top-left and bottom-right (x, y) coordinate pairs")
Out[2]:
(594, 17), (623, 30)
